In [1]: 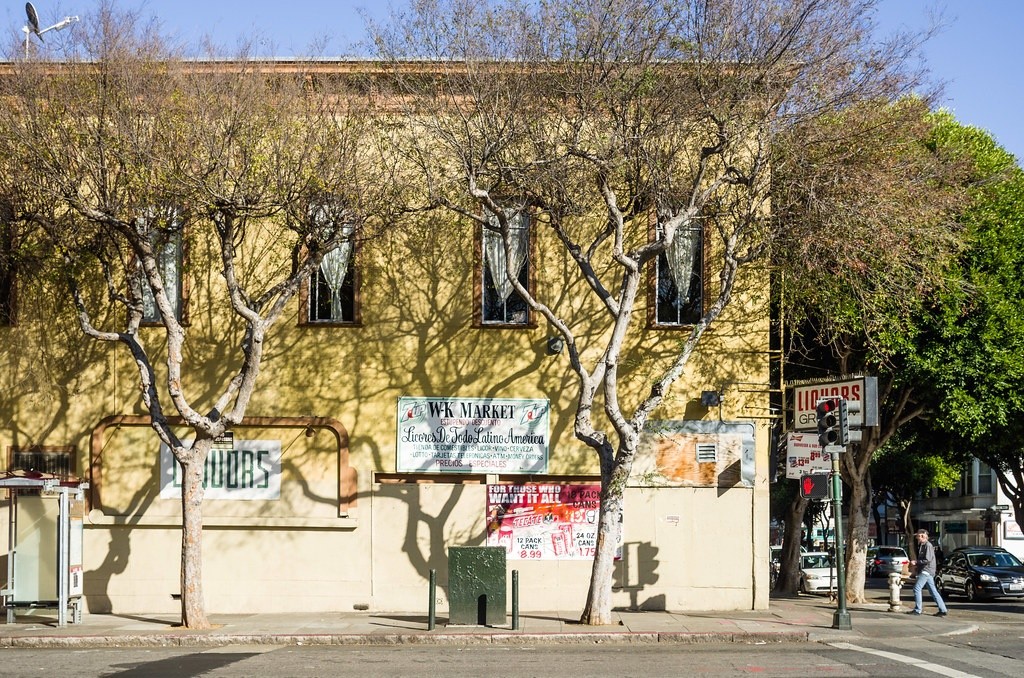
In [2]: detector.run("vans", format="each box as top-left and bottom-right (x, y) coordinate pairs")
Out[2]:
(866, 546), (910, 579)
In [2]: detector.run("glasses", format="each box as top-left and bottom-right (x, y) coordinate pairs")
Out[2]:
(918, 535), (924, 538)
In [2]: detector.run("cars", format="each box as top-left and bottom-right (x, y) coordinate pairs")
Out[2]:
(798, 552), (838, 594)
(769, 545), (808, 569)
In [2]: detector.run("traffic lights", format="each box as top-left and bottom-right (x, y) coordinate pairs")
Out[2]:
(800, 475), (828, 499)
(817, 400), (837, 445)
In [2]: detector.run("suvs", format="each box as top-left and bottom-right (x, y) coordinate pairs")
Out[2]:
(935, 545), (1024, 602)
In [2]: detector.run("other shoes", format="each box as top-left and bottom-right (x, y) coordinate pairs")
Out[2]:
(933, 611), (946, 617)
(906, 610), (919, 615)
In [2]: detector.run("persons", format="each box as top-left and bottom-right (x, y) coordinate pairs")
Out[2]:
(935, 546), (944, 566)
(980, 557), (990, 566)
(904, 528), (949, 617)
